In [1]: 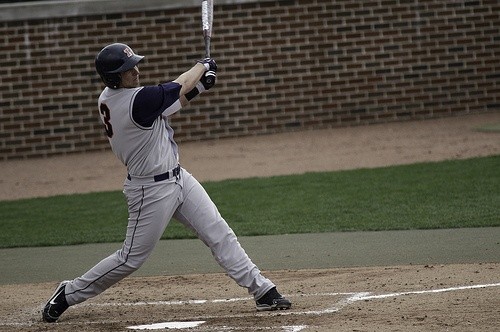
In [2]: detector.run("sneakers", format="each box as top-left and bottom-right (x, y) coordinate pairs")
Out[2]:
(256, 286), (291, 311)
(42, 280), (69, 323)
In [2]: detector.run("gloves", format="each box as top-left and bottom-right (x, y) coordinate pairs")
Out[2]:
(199, 70), (216, 90)
(195, 57), (217, 72)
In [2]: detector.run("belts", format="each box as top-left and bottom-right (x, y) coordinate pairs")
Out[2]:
(127, 165), (180, 182)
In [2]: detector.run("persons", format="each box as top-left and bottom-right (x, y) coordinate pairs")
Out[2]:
(41, 42), (292, 322)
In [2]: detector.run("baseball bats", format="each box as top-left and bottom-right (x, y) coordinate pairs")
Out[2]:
(201, 0), (217, 87)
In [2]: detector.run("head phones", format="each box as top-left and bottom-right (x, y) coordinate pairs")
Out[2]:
(101, 73), (121, 89)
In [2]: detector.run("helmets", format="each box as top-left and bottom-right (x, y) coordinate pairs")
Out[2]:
(95, 43), (145, 89)
